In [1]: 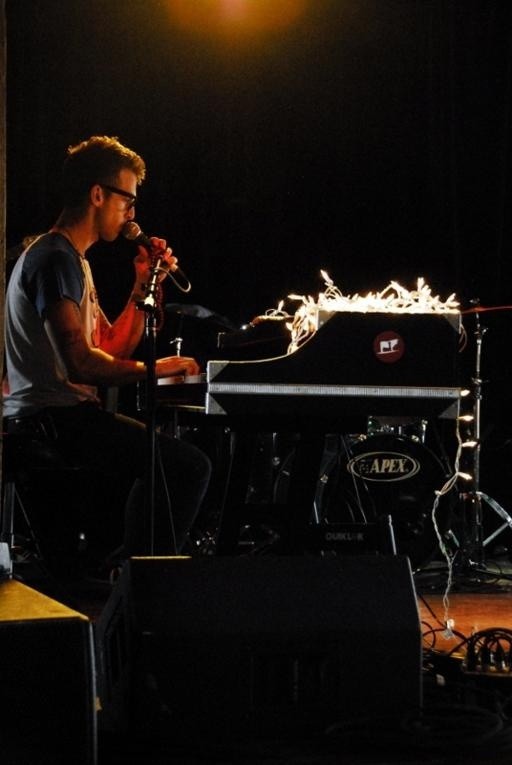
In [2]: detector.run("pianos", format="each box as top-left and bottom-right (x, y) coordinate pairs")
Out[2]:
(131, 313), (461, 556)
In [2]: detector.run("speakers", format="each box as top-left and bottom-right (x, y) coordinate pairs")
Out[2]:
(96, 555), (425, 739)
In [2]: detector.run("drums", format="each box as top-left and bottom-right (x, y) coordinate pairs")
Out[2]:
(314, 423), (459, 575)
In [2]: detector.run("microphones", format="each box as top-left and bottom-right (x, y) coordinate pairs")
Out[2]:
(123, 222), (185, 278)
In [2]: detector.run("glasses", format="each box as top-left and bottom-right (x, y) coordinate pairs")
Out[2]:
(100, 184), (137, 211)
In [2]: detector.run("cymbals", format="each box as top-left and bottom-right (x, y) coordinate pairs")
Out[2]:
(461, 306), (510, 315)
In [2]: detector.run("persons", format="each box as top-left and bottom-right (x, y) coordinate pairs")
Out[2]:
(4, 136), (212, 553)
